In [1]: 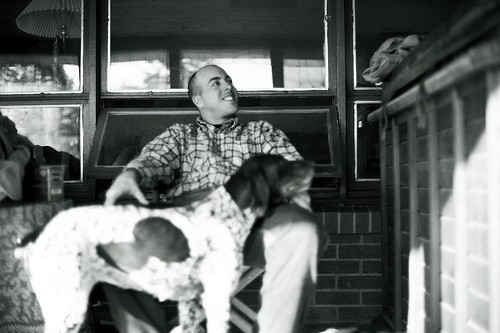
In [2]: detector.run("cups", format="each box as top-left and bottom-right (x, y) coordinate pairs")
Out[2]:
(39, 165), (66, 202)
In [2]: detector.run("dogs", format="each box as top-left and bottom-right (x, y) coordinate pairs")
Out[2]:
(14, 153), (315, 333)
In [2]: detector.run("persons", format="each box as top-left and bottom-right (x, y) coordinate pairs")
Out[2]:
(100, 65), (323, 333)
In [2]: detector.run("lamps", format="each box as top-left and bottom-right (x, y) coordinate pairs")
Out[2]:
(15, 0), (81, 56)
